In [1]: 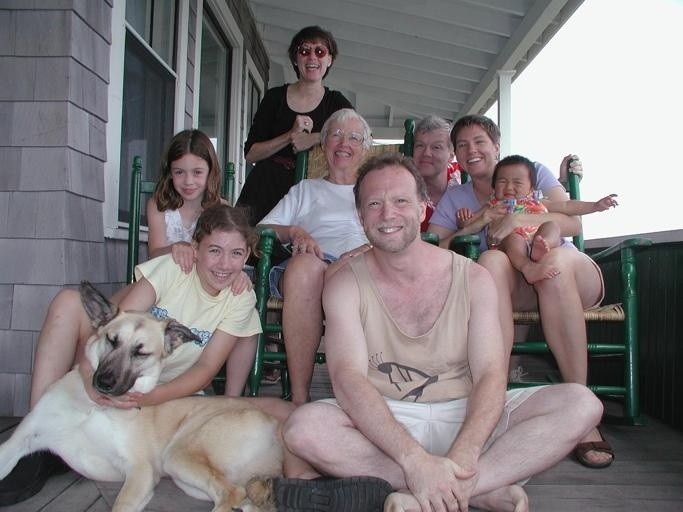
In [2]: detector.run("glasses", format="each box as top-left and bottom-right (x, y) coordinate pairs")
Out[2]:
(299, 45), (327, 58)
(328, 128), (364, 146)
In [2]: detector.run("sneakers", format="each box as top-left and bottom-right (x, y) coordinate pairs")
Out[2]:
(0, 451), (71, 507)
(271, 476), (393, 511)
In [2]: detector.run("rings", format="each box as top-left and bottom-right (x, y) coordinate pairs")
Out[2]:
(304, 121), (310, 126)
(348, 253), (354, 257)
(290, 245), (299, 249)
(490, 244), (496, 246)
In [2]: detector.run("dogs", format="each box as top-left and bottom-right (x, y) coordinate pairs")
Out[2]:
(0, 278), (285, 512)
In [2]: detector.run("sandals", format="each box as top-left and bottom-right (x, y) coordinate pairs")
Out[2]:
(575, 428), (614, 468)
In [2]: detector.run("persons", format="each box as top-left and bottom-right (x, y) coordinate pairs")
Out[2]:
(2, 200), (397, 512)
(280, 151), (607, 512)
(425, 113), (617, 468)
(231, 22), (353, 387)
(253, 107), (375, 408)
(143, 129), (263, 397)
(404, 115), (584, 230)
(455, 154), (618, 283)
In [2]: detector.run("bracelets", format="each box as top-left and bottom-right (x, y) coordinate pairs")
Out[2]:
(363, 241), (374, 249)
